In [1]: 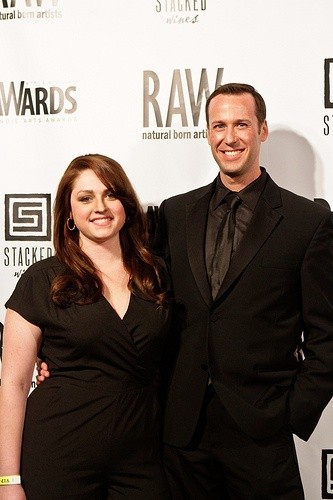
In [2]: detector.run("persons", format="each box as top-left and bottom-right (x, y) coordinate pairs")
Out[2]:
(1, 154), (172, 500)
(143, 82), (333, 500)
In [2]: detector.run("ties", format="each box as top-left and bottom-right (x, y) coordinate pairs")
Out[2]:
(207, 193), (242, 296)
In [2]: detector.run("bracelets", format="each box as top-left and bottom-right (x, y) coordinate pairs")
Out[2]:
(0, 476), (21, 486)
